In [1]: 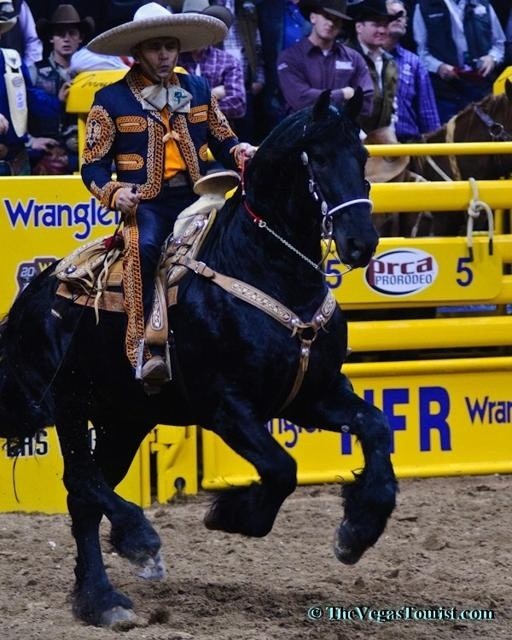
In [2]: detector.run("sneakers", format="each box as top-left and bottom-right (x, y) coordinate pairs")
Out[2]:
(135, 354), (166, 388)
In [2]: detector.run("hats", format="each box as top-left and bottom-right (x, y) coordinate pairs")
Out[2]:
(87, 2), (228, 56)
(297, 0), (353, 28)
(178, 0), (233, 29)
(347, 0), (404, 23)
(36, 5), (95, 36)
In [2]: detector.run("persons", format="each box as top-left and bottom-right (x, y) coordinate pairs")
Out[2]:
(386, 0), (441, 142)
(0, 48), (31, 176)
(177, 5), (247, 123)
(23, 4), (94, 176)
(81, 2), (249, 377)
(345, 0), (404, 138)
(411, 1), (506, 116)
(0, 1), (43, 68)
(276, 0), (377, 136)
(181, 0), (265, 94)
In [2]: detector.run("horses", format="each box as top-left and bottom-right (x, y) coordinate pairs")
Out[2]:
(1, 86), (398, 627)
(347, 79), (511, 360)
